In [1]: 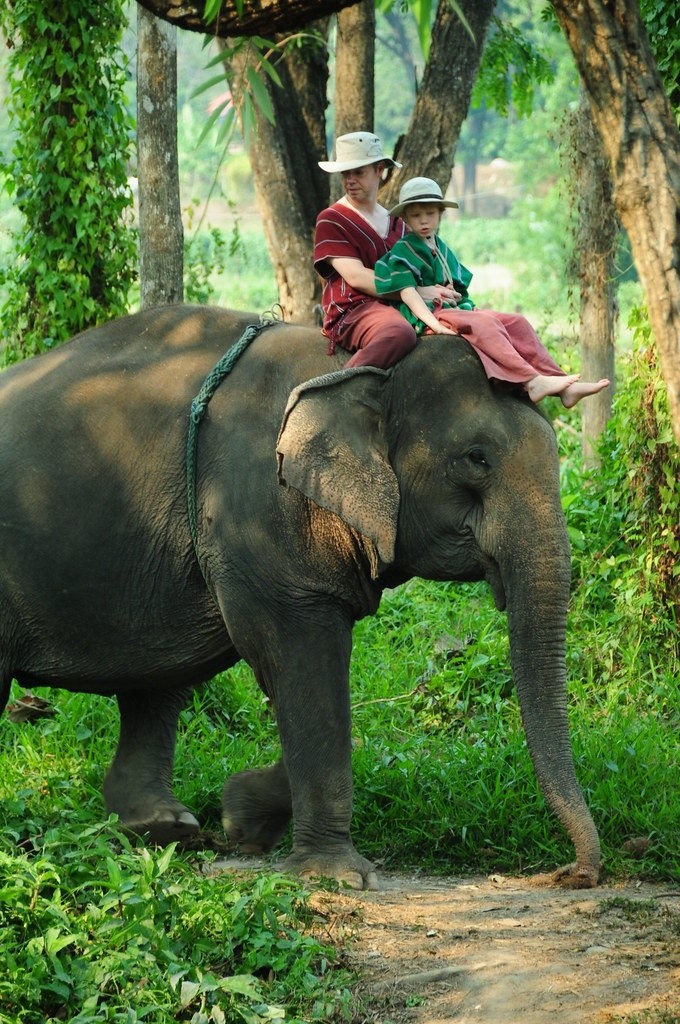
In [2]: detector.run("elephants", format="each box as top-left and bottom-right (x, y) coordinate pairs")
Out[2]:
(0, 303), (602, 893)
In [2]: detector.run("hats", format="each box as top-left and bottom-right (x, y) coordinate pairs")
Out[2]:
(387, 176), (458, 215)
(317, 132), (403, 173)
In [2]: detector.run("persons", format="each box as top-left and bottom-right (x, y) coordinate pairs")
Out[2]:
(313, 132), (611, 409)
